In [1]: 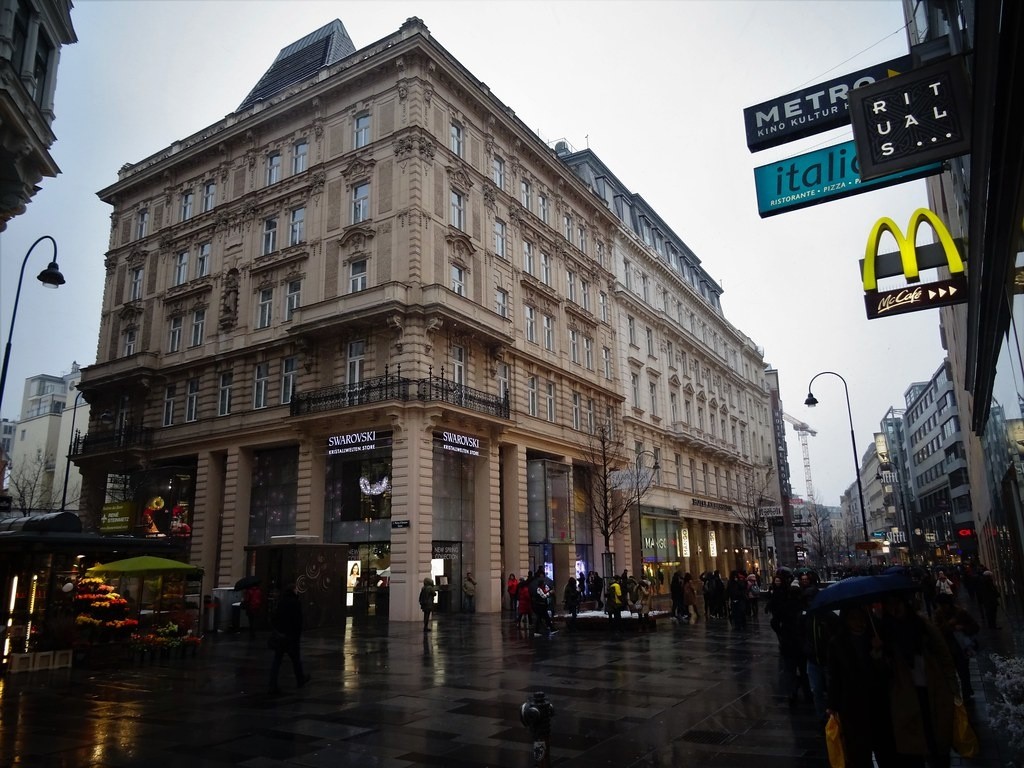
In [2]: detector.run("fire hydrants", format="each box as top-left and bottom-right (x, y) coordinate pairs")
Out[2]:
(519, 691), (555, 768)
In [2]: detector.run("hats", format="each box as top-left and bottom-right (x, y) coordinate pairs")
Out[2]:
(746, 573), (757, 581)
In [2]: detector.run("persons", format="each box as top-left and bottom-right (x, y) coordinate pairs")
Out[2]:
(699, 566), (761, 630)
(607, 575), (652, 641)
(564, 577), (578, 632)
(908, 563), (1003, 631)
(243, 583), (262, 640)
(577, 571), (604, 610)
(764, 565), (869, 716)
(267, 584), (311, 696)
(828, 590), (954, 768)
(933, 595), (980, 700)
(621, 569), (628, 580)
(419, 578), (436, 632)
(507, 566), (560, 636)
(461, 572), (477, 613)
(669, 570), (701, 620)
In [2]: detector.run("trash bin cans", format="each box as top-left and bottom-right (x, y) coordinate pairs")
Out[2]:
(204, 594), (221, 633)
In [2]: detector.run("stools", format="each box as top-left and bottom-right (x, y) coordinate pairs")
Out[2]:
(33, 650), (54, 671)
(6, 652), (35, 673)
(53, 649), (73, 670)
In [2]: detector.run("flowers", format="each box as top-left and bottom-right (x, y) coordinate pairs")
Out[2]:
(76, 571), (202, 652)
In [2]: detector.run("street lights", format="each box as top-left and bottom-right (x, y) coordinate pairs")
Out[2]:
(635, 451), (660, 578)
(806, 372), (874, 567)
(898, 494), (938, 578)
(746, 488), (765, 574)
(817, 518), (834, 578)
(0, 235), (65, 398)
(875, 461), (918, 585)
(799, 508), (814, 567)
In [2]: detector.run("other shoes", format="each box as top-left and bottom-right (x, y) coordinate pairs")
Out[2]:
(549, 626), (560, 634)
(670, 617), (678, 620)
(710, 615), (716, 619)
(716, 617), (719, 620)
(424, 627), (431, 633)
(682, 615), (688, 620)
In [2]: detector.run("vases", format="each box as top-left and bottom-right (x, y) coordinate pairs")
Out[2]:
(143, 648), (151, 658)
(151, 645), (162, 657)
(181, 644), (196, 655)
(161, 647), (167, 656)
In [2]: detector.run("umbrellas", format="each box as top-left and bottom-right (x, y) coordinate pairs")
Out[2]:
(235, 576), (261, 592)
(86, 556), (204, 602)
(883, 566), (903, 574)
(810, 574), (919, 633)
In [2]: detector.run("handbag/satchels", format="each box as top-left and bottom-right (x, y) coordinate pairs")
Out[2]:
(952, 704), (979, 758)
(750, 581), (760, 595)
(630, 586), (638, 603)
(823, 711), (845, 768)
(635, 600), (643, 610)
